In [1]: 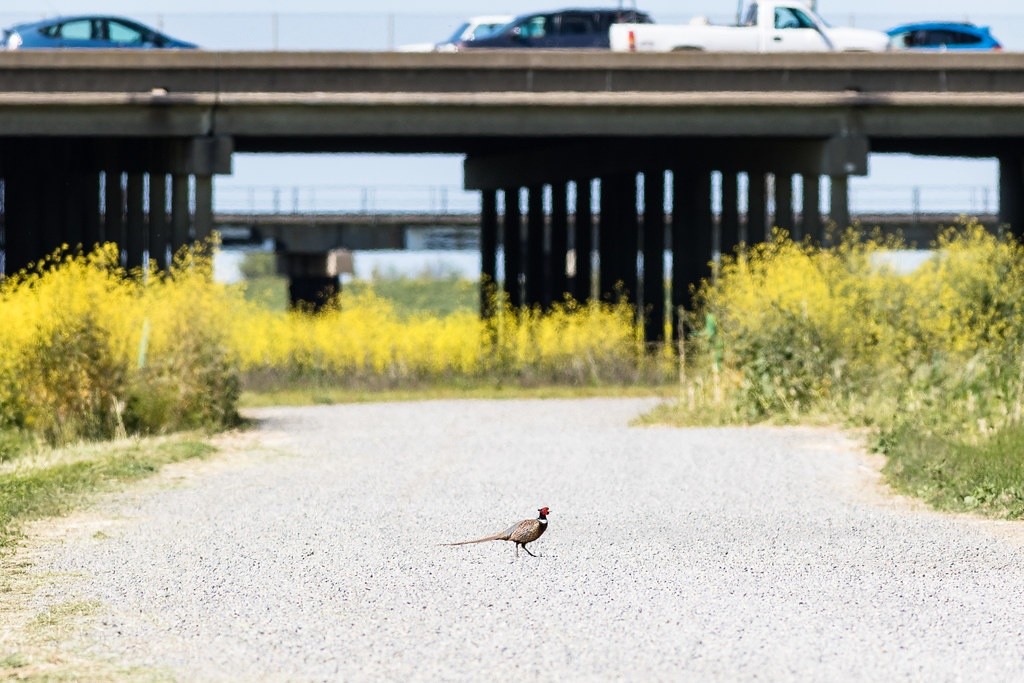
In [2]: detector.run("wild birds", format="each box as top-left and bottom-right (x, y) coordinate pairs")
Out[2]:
(441, 506), (550, 557)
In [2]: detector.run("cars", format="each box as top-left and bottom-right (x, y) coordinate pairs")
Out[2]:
(883, 20), (1001, 51)
(430, 3), (657, 54)
(0, 12), (203, 50)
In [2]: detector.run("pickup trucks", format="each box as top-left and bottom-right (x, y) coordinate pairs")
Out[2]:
(608, 0), (895, 56)
